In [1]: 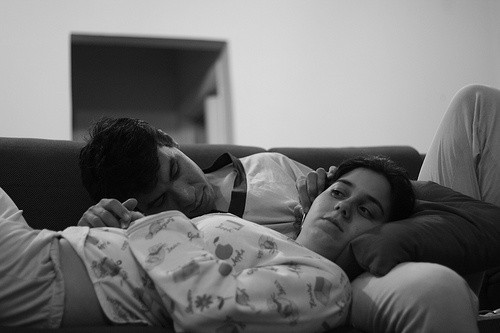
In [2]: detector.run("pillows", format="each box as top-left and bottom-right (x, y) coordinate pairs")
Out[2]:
(294, 178), (499, 277)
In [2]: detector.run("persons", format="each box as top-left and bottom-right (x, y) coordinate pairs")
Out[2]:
(77, 83), (500, 241)
(0, 154), (416, 333)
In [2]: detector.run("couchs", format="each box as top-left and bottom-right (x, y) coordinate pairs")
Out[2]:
(0, 137), (500, 333)
